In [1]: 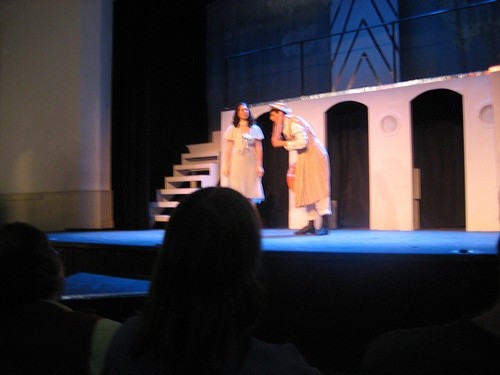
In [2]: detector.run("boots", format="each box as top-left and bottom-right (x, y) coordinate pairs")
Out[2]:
(314, 214), (330, 236)
(293, 221), (316, 237)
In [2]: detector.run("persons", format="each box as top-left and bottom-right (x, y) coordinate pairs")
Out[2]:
(0, 187), (500, 375)
(223, 103), (265, 232)
(269, 103), (332, 236)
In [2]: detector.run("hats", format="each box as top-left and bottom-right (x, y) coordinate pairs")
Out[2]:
(268, 101), (292, 115)
